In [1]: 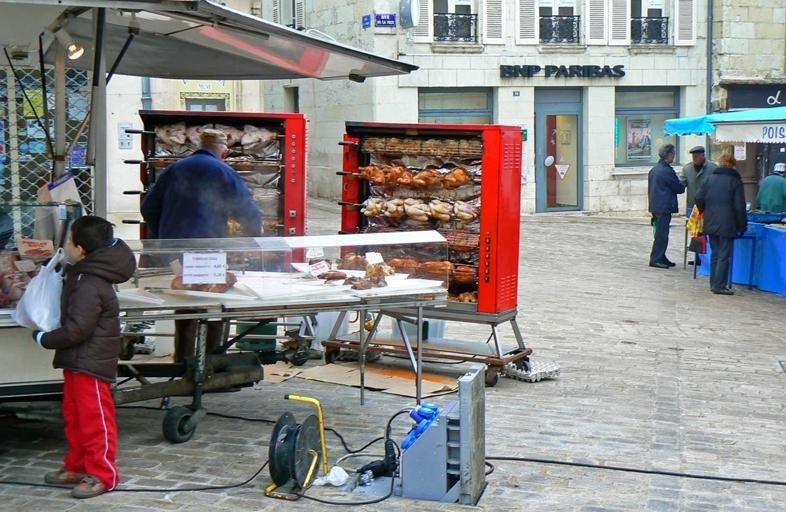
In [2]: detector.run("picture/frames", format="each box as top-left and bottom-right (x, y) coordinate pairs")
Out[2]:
(625, 116), (653, 162)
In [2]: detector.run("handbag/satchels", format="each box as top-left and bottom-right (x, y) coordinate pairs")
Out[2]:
(689, 236), (707, 254)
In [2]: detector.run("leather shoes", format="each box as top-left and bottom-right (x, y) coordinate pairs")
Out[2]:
(713, 287), (734, 295)
(662, 258), (676, 267)
(649, 261), (669, 268)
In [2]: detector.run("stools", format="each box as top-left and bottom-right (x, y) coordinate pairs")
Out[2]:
(692, 231), (757, 289)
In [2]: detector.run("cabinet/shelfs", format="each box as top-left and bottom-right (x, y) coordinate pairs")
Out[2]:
(113, 229), (449, 409)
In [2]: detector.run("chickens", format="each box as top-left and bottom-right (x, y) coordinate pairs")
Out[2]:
(226, 214), (281, 238)
(171, 270), (238, 293)
(153, 123), (278, 154)
(337, 135), (481, 303)
(227, 251), (276, 267)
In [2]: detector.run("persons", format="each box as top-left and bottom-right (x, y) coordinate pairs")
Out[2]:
(755, 162), (786, 213)
(695, 153), (747, 295)
(32, 217), (135, 499)
(141, 129), (263, 361)
(679, 146), (719, 265)
(648, 144), (685, 268)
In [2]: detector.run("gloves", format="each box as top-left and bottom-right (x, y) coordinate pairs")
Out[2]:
(41, 257), (65, 278)
(32, 330), (46, 348)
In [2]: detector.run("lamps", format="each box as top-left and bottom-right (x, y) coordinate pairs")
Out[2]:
(52, 26), (84, 61)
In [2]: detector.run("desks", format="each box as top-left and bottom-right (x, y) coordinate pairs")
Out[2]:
(682, 208), (786, 297)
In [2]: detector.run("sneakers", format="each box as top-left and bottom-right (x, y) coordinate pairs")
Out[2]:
(71, 474), (108, 498)
(43, 470), (81, 484)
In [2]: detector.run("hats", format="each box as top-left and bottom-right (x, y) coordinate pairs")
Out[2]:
(773, 163), (786, 172)
(688, 146), (705, 153)
(199, 129), (228, 144)
